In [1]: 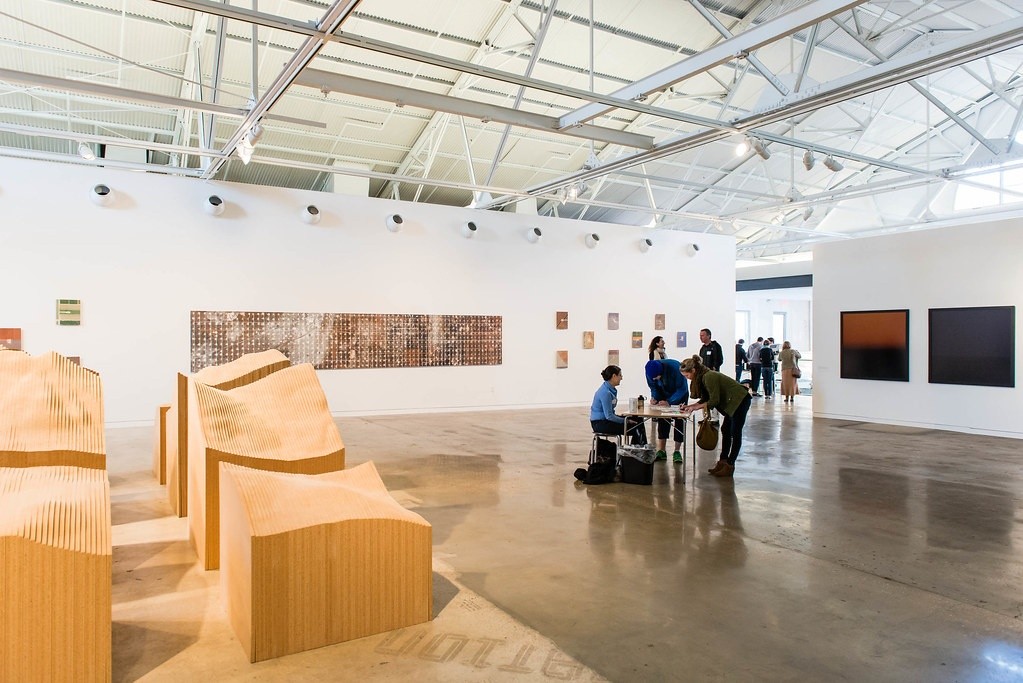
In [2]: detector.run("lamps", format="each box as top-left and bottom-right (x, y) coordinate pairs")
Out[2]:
(736, 133), (772, 161)
(803, 149), (816, 170)
(824, 154), (843, 172)
(236, 124), (265, 166)
(78, 140), (95, 161)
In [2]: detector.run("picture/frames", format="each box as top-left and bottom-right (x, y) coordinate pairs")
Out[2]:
(840, 309), (909, 382)
(928, 306), (1015, 387)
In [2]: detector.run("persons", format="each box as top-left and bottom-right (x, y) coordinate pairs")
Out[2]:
(590, 365), (647, 447)
(679, 356), (753, 477)
(736, 337), (801, 402)
(649, 336), (668, 447)
(698, 329), (723, 427)
(645, 358), (689, 462)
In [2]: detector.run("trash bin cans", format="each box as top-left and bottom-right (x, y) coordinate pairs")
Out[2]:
(618, 444), (655, 485)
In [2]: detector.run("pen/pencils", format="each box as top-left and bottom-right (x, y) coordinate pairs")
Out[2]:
(678, 402), (685, 410)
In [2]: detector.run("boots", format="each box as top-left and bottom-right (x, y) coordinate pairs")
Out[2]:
(708, 460), (728, 474)
(715, 463), (734, 477)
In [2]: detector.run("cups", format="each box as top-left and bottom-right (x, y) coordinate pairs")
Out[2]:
(629, 398), (638, 413)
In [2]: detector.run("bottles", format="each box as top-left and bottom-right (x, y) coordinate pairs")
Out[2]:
(638, 395), (645, 409)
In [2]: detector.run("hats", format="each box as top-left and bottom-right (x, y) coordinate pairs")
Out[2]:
(645, 361), (663, 379)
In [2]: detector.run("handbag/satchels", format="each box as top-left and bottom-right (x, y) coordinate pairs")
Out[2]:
(696, 404), (718, 450)
(596, 437), (617, 466)
(792, 366), (801, 379)
(585, 463), (614, 485)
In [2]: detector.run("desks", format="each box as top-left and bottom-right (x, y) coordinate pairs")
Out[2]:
(622, 407), (695, 486)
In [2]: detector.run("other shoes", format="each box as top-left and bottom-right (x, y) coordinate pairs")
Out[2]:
(765, 395), (772, 400)
(790, 398), (793, 402)
(752, 392), (763, 397)
(784, 399), (788, 402)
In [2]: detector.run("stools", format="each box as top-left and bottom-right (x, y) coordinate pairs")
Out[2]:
(591, 431), (622, 471)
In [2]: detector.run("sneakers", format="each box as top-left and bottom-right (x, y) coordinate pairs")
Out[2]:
(673, 452), (682, 463)
(655, 450), (668, 461)
